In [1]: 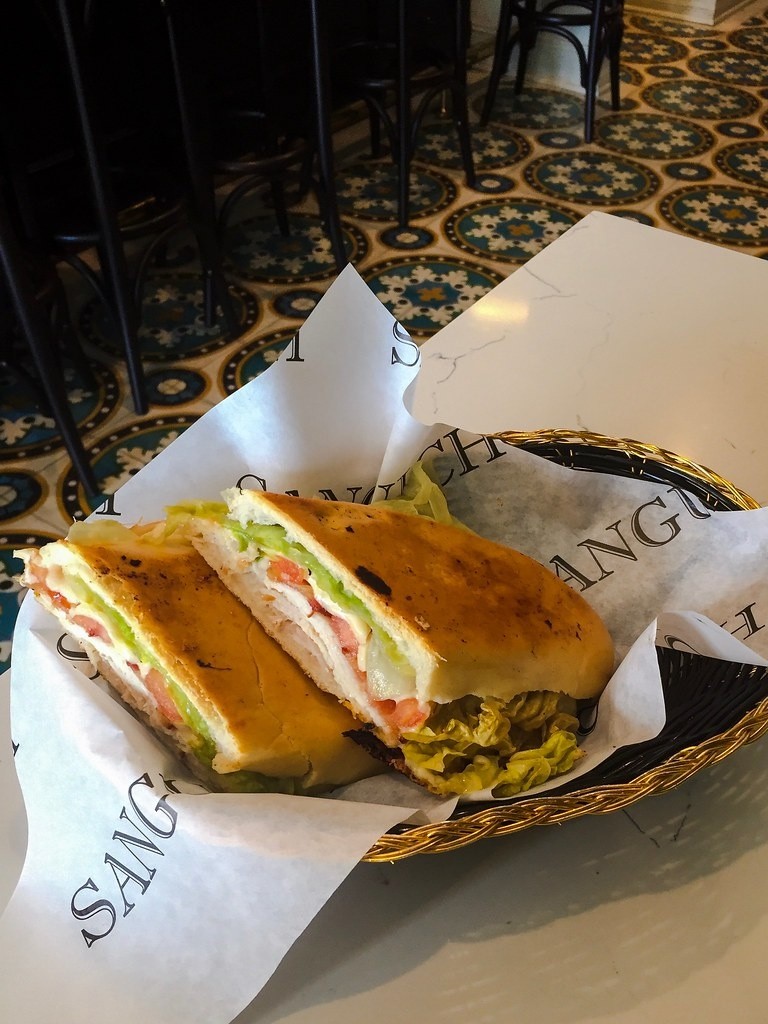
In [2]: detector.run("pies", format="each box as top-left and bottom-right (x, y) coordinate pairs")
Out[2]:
(180, 460), (618, 800)
(8, 518), (391, 797)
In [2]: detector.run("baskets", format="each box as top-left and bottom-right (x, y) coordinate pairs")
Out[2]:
(186, 428), (768, 863)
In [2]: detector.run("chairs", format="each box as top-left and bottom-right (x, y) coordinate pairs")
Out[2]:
(1, 0), (625, 494)
